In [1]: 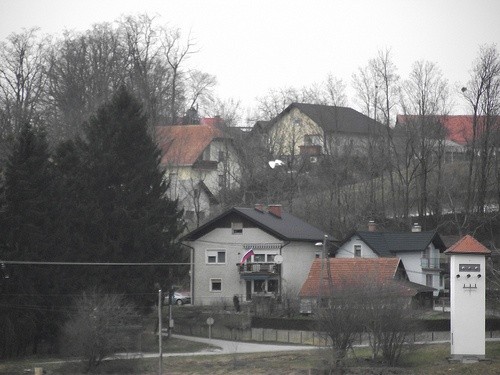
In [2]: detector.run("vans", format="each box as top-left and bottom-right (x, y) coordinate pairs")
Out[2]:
(300, 298), (318, 315)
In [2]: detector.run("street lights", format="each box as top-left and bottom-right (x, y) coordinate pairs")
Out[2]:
(315, 234), (330, 282)
(268, 154), (318, 215)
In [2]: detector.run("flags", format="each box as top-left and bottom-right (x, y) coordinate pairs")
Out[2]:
(239, 248), (255, 269)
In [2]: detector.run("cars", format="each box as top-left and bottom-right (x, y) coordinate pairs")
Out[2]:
(163, 291), (192, 306)
